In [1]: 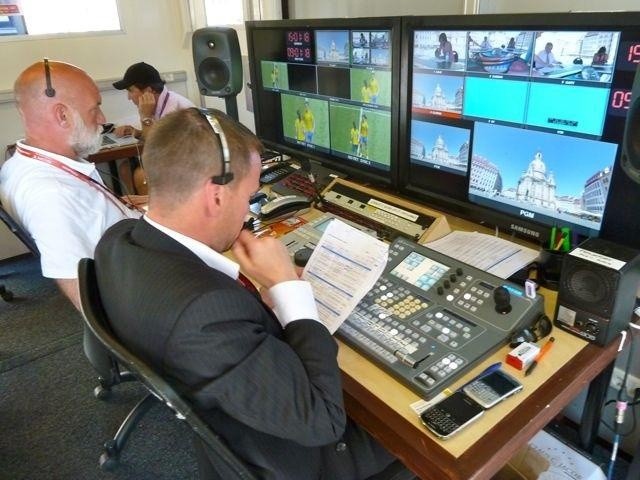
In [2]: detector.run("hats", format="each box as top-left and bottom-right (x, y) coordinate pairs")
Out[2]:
(113, 62), (160, 89)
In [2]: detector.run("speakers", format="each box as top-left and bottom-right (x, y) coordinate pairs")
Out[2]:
(620, 65), (639, 183)
(191, 27), (242, 98)
(553, 239), (640, 346)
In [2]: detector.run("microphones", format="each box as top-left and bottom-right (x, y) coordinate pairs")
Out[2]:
(240, 216), (256, 234)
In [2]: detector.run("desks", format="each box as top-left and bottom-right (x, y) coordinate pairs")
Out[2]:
(222, 159), (640, 480)
(7, 124), (143, 197)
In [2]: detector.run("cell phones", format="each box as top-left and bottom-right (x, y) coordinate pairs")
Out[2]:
(464, 371), (524, 409)
(418, 392), (486, 439)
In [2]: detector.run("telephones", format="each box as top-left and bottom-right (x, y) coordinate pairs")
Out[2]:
(258, 195), (313, 221)
(259, 162), (301, 183)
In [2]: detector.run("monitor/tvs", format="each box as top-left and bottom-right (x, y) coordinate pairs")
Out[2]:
(244, 15), (399, 189)
(400, 11), (640, 244)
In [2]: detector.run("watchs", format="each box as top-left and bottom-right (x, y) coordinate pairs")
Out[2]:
(139, 117), (153, 126)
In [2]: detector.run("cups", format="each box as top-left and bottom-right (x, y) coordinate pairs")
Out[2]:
(541, 249), (563, 291)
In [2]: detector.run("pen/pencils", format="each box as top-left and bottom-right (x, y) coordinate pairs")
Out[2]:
(550, 220), (577, 251)
(457, 362), (502, 391)
(525, 337), (555, 377)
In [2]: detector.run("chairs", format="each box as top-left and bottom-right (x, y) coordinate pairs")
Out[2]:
(0, 201), (138, 400)
(79, 258), (258, 480)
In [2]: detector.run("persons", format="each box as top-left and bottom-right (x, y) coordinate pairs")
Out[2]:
(94, 107), (417, 480)
(592, 46), (609, 65)
(468, 32), (516, 51)
(112, 61), (197, 195)
(270, 64), (379, 159)
(437, 33), (455, 63)
(535, 42), (562, 71)
(0, 60), (148, 312)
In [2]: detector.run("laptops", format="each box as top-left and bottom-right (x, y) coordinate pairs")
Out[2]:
(99, 134), (138, 149)
